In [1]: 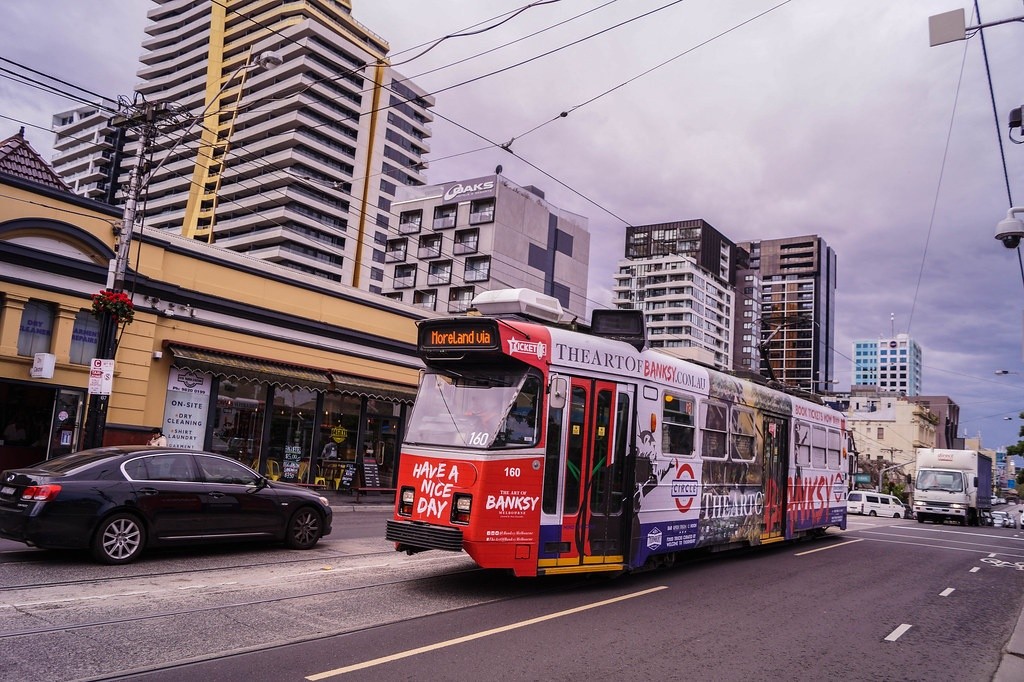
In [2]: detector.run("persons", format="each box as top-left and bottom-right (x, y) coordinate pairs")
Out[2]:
(146, 427), (167, 447)
(535, 415), (561, 502)
(668, 411), (694, 455)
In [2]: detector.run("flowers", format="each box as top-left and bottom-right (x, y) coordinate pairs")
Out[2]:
(90, 290), (135, 325)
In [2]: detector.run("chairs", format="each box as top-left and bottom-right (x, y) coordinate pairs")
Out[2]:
(251, 459), (258, 471)
(315, 464), (326, 490)
(266, 459), (282, 482)
(298, 462), (307, 483)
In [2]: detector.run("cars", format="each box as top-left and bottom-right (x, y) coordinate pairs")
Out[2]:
(212, 436), (253, 453)
(990, 496), (1024, 530)
(0, 446), (333, 564)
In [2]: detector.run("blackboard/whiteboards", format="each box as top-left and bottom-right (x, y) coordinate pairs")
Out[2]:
(339, 463), (359, 489)
(283, 445), (301, 481)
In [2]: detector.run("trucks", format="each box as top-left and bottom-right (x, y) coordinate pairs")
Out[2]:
(913, 448), (992, 526)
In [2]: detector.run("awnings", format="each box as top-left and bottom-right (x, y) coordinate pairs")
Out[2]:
(328, 372), (417, 408)
(164, 345), (334, 396)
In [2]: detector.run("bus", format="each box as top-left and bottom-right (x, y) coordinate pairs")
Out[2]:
(385, 288), (858, 577)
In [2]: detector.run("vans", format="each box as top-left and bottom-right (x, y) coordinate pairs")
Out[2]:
(846, 491), (906, 518)
